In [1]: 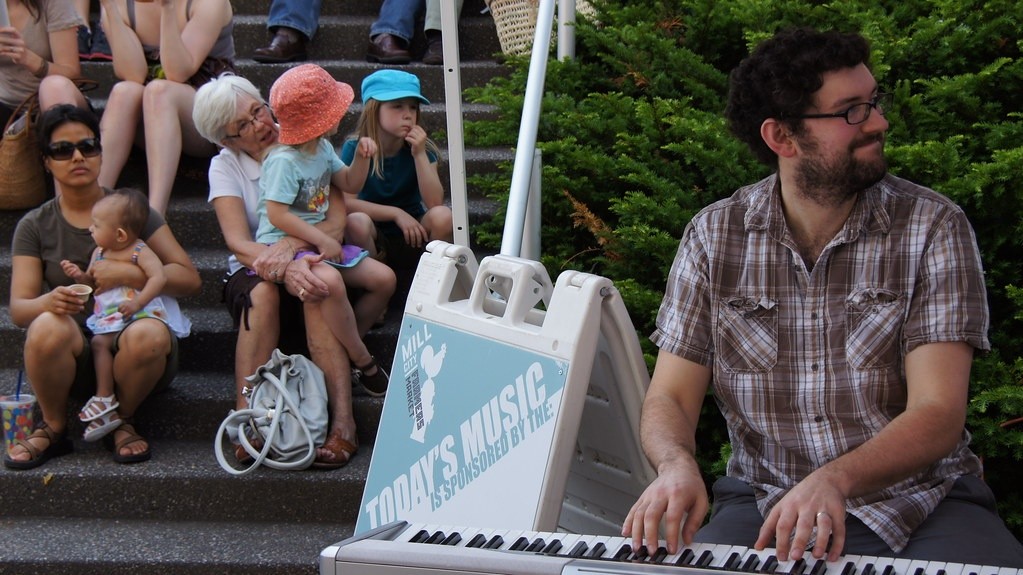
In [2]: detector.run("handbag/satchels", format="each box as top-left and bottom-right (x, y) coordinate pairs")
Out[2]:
(1, 77), (98, 210)
(139, 46), (245, 91)
(213, 348), (329, 476)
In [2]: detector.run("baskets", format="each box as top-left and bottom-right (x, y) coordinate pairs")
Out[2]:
(486, 0), (604, 59)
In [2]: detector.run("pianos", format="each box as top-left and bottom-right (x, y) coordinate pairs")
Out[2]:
(320, 520), (1023, 575)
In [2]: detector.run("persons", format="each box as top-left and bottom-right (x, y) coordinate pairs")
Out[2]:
(0, 0), (467, 473)
(624, 30), (1023, 569)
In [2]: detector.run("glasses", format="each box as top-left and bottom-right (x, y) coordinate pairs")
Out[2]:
(789, 92), (894, 125)
(46, 136), (102, 161)
(225, 101), (270, 140)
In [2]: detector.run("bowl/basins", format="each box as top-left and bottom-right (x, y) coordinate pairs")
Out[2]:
(68, 284), (92, 302)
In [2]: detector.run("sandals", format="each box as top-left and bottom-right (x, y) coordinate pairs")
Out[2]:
(103, 416), (151, 462)
(82, 411), (122, 443)
(312, 433), (360, 469)
(231, 434), (261, 464)
(4, 420), (74, 469)
(79, 394), (119, 422)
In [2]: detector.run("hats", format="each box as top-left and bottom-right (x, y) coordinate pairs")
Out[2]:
(270, 63), (355, 146)
(361, 69), (431, 107)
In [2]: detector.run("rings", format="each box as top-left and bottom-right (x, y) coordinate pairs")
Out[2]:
(298, 288), (304, 302)
(816, 511), (833, 521)
(269, 270), (278, 276)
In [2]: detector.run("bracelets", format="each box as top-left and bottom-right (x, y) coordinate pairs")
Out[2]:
(32, 58), (49, 78)
(281, 238), (296, 259)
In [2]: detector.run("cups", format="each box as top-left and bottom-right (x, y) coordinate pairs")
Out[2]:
(0, 394), (37, 454)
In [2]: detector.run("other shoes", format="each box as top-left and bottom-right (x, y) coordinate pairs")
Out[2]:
(423, 32), (443, 64)
(349, 354), (389, 397)
(75, 20), (96, 52)
(372, 305), (394, 323)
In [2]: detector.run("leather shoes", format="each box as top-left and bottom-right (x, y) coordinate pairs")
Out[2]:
(253, 33), (308, 61)
(367, 34), (411, 63)
